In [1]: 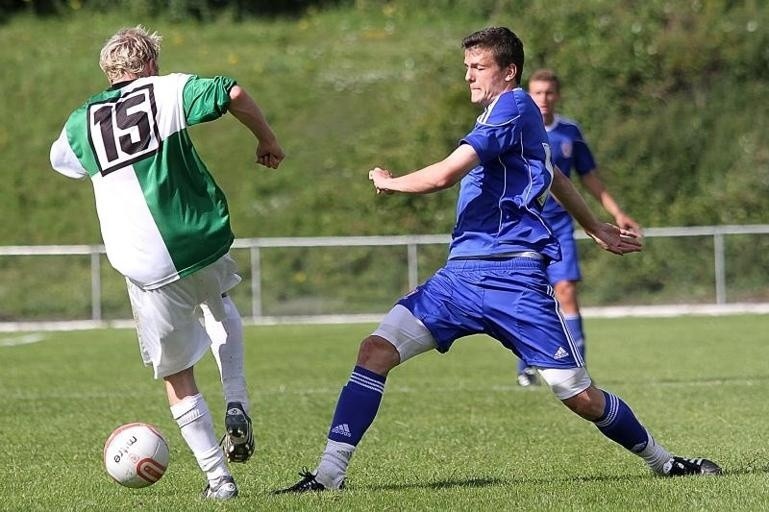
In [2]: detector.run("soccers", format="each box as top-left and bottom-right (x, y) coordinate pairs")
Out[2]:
(103, 423), (169, 488)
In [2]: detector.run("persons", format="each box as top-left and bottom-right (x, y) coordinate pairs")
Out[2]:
(49, 25), (285, 503)
(518, 69), (641, 387)
(276, 26), (723, 492)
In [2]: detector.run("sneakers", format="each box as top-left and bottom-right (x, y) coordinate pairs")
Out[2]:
(201, 475), (238, 500)
(275, 466), (345, 494)
(218, 401), (255, 465)
(663, 455), (722, 477)
